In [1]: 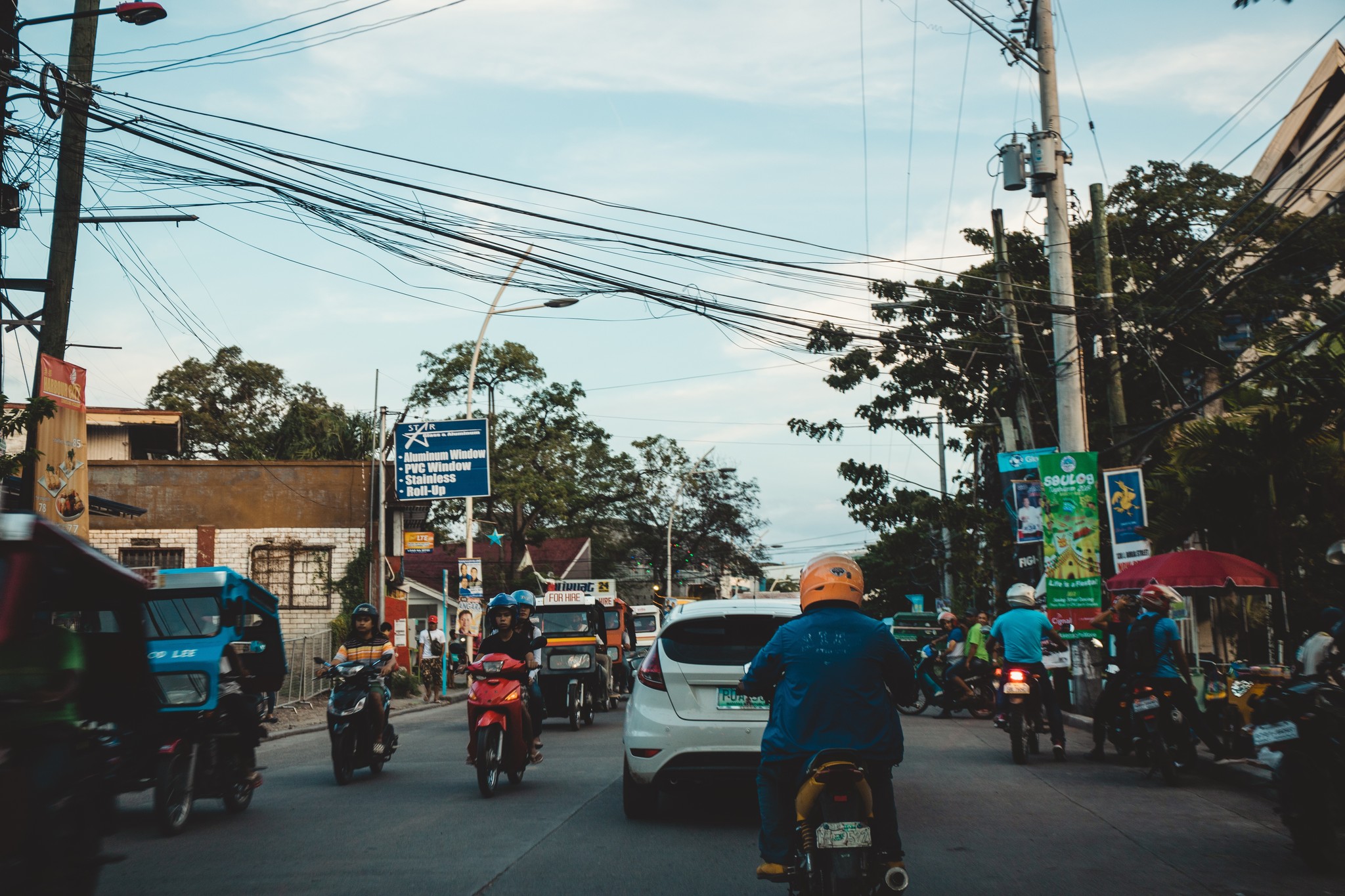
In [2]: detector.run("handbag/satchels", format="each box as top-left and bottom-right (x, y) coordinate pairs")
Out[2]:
(428, 629), (442, 656)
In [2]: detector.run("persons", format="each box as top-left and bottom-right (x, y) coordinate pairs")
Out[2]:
(562, 612), (609, 712)
(490, 589), (545, 750)
(454, 593), (544, 765)
(378, 620), (397, 710)
(976, 611), (998, 672)
(634, 617), (656, 632)
(313, 601), (397, 756)
(604, 611), (632, 693)
(418, 615), (446, 705)
(472, 624), (483, 662)
(1082, 591), (1142, 763)
(457, 563), (482, 635)
(929, 611), (966, 720)
(663, 600), (676, 617)
(736, 554), (924, 882)
(1126, 584), (1253, 774)
(447, 628), (460, 688)
(944, 607), (990, 705)
(1294, 607), (1345, 709)
(985, 582), (1069, 761)
(596, 652), (621, 699)
(1015, 497), (1098, 581)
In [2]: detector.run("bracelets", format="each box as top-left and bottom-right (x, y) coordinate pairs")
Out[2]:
(321, 667), (329, 671)
(992, 659), (997, 662)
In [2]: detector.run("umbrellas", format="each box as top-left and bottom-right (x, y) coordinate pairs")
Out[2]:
(1105, 547), (1281, 679)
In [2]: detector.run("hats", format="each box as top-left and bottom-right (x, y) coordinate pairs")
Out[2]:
(428, 615), (438, 624)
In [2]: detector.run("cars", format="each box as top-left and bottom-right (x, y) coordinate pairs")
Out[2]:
(889, 611), (946, 666)
(620, 597), (804, 820)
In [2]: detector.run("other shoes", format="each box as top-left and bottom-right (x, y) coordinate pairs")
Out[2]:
(756, 862), (788, 875)
(887, 860), (906, 870)
(373, 743), (385, 754)
(264, 717), (278, 723)
(608, 687), (629, 698)
(245, 771), (263, 786)
(932, 694), (1248, 765)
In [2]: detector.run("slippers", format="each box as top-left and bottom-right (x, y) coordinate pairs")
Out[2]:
(528, 751), (544, 764)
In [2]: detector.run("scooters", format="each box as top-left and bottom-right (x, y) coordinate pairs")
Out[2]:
(312, 650), (400, 787)
(447, 635), (547, 801)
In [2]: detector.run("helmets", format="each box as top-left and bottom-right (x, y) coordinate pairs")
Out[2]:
(937, 611), (958, 628)
(1006, 583), (1037, 607)
(1140, 583), (1185, 611)
(352, 603), (378, 616)
(800, 551), (865, 613)
(510, 590), (536, 613)
(486, 592), (521, 620)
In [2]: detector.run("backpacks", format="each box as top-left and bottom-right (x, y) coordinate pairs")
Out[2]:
(1124, 614), (1170, 676)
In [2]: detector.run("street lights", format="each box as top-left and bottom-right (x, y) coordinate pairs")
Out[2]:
(465, 242), (579, 698)
(667, 447), (737, 599)
(817, 411), (955, 600)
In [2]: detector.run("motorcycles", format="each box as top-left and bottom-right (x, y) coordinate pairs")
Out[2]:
(142, 567), (287, 838)
(1249, 620), (1345, 880)
(593, 596), (638, 713)
(628, 605), (661, 652)
(1090, 637), (1295, 788)
(980, 622), (1075, 766)
(739, 658), (909, 896)
(895, 630), (998, 720)
(489, 589), (612, 733)
(0, 512), (166, 896)
